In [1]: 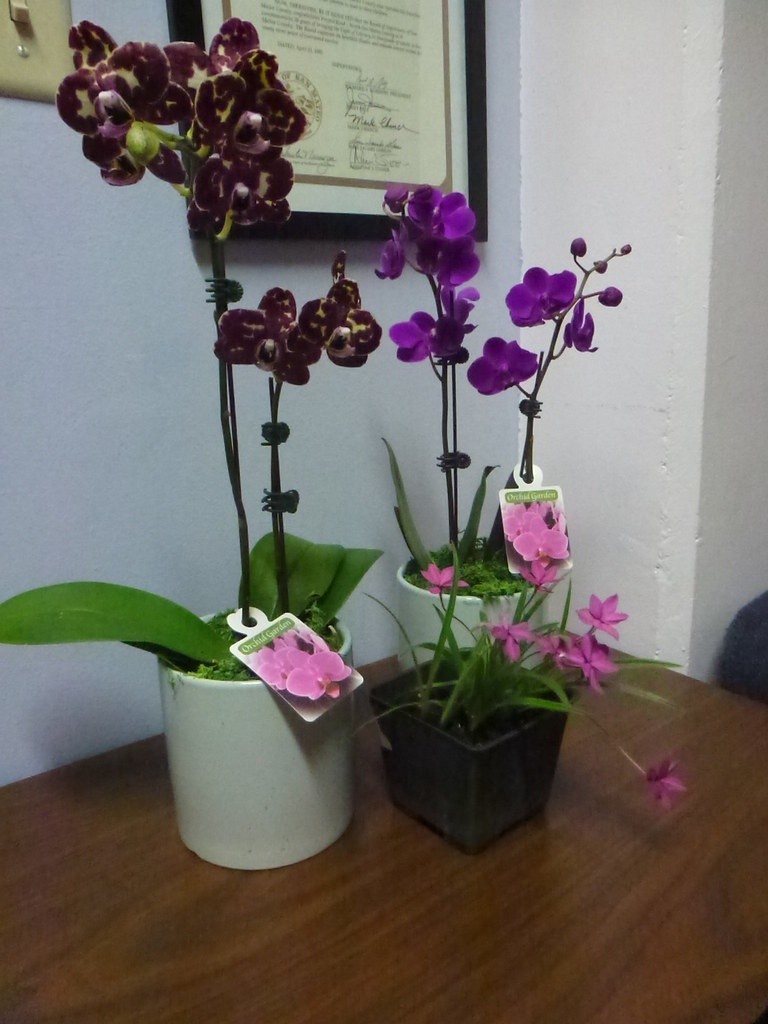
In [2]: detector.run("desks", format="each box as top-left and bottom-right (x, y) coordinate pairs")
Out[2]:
(1, 639), (768, 1024)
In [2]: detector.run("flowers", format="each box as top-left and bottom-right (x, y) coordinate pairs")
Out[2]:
(503, 501), (571, 578)
(0, 14), (685, 812)
(255, 630), (353, 701)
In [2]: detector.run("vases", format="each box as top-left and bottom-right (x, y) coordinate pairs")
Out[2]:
(157, 614), (359, 871)
(368, 653), (576, 855)
(398, 557), (552, 670)
